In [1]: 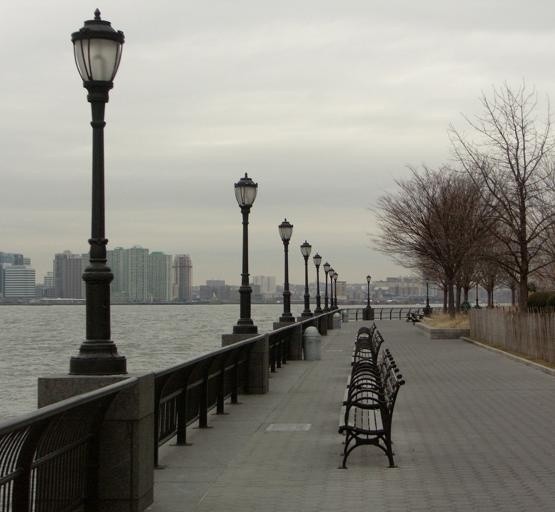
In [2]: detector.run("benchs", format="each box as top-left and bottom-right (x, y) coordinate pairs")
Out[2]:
(337, 322), (405, 468)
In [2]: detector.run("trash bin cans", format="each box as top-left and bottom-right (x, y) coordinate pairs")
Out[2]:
(303, 326), (322, 361)
(333, 312), (342, 329)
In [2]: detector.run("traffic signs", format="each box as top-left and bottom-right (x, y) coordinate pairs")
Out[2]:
(70, 8), (126, 375)
(426, 278), (430, 308)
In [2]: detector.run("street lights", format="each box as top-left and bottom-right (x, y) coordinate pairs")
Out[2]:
(366, 276), (371, 310)
(278, 219), (295, 323)
(233, 173), (257, 334)
(300, 241), (338, 316)
